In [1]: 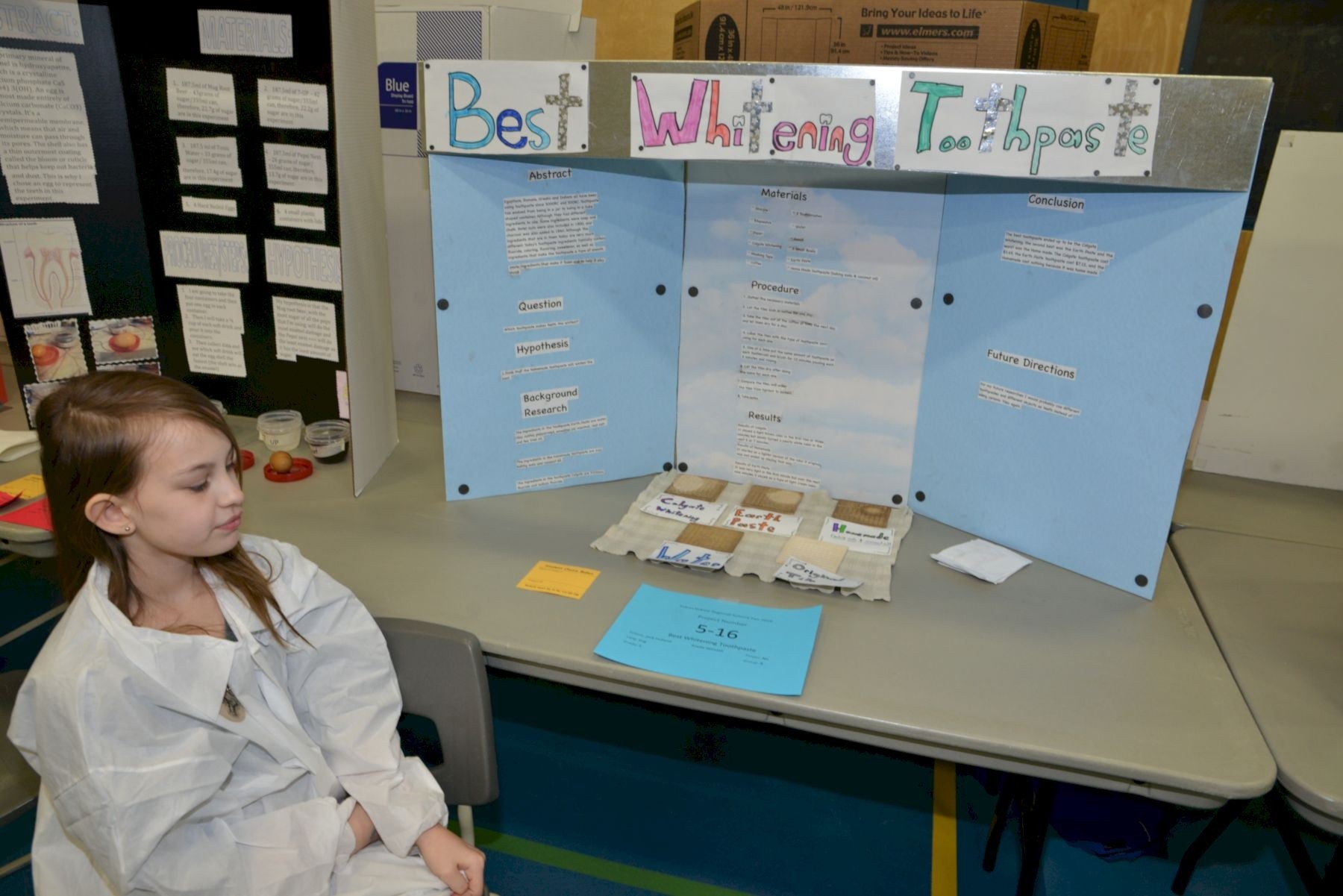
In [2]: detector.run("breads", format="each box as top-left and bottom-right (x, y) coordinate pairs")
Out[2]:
(114, 333), (136, 347)
(31, 344), (46, 357)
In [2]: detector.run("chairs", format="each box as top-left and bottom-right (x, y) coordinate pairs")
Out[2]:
(375, 617), (494, 846)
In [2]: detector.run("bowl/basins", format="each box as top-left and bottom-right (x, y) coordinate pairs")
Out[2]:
(54, 330), (76, 348)
(107, 319), (130, 334)
(304, 419), (350, 464)
(256, 409), (303, 452)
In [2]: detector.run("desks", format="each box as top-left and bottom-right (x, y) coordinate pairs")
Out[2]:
(0, 360), (1343, 896)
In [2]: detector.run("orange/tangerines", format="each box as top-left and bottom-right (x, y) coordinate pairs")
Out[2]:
(269, 451), (293, 473)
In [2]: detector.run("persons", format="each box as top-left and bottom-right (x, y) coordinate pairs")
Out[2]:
(6, 372), (485, 896)
